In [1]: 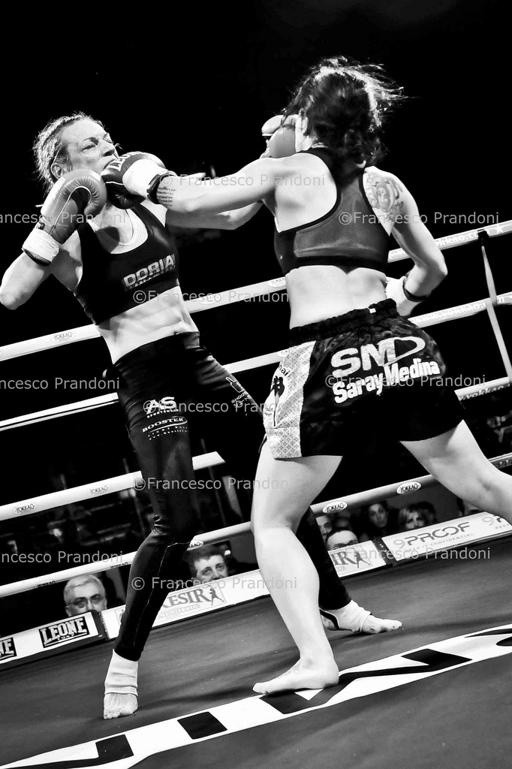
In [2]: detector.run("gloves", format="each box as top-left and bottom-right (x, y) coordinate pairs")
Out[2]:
(260, 110), (296, 158)
(101, 151), (176, 209)
(21, 169), (107, 266)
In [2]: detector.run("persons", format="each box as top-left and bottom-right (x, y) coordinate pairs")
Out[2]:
(2, 110), (402, 722)
(119, 47), (512, 698)
